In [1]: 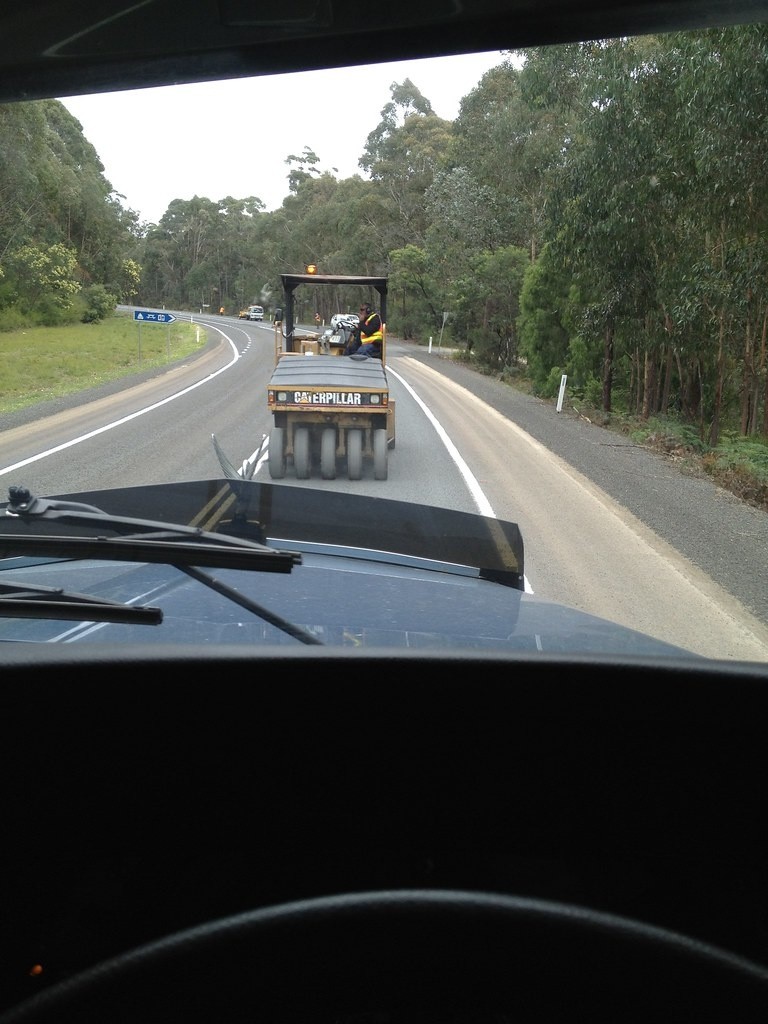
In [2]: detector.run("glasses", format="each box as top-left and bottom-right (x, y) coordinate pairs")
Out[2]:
(360, 308), (366, 312)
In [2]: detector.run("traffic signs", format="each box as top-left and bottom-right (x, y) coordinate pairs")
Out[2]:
(133, 311), (178, 324)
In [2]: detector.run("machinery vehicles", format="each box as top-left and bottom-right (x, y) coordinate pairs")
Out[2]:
(266, 265), (395, 480)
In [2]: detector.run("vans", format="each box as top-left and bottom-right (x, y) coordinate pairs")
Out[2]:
(247, 305), (264, 321)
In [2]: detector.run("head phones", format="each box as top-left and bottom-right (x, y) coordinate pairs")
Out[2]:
(366, 302), (372, 314)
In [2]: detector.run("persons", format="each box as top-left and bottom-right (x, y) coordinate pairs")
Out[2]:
(315, 313), (321, 329)
(344, 302), (383, 356)
(220, 306), (225, 316)
